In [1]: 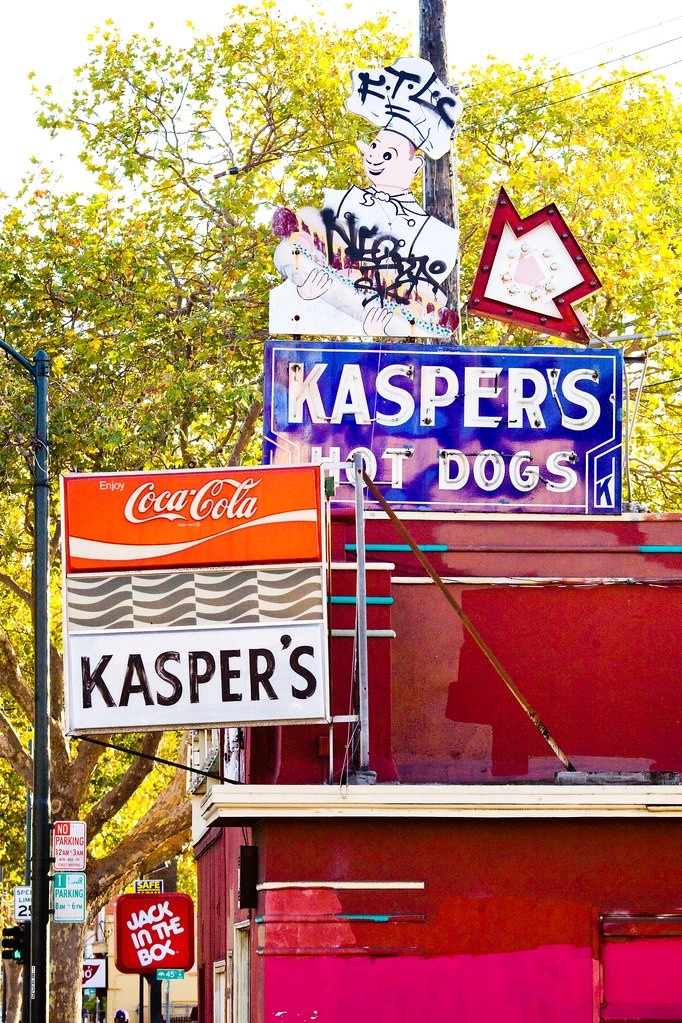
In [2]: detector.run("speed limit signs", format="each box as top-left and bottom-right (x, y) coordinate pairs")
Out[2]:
(12, 885), (32, 922)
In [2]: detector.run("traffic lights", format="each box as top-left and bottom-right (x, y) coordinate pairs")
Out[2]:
(1, 925), (25, 961)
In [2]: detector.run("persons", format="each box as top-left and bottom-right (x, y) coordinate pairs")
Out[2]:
(114, 1009), (129, 1023)
